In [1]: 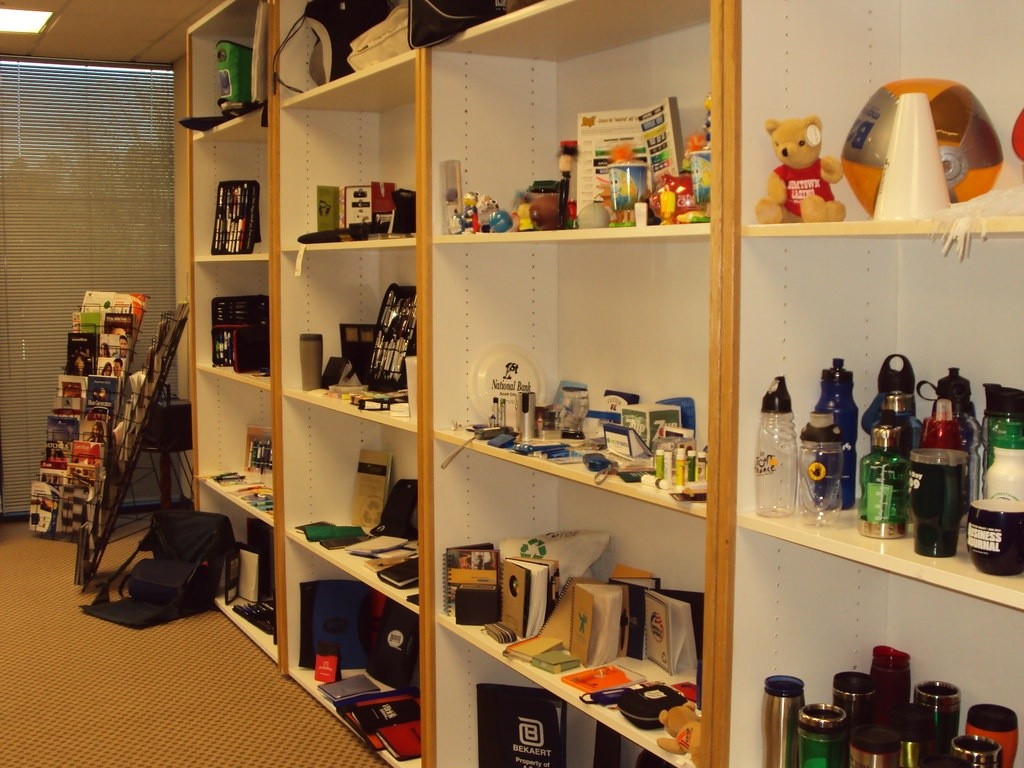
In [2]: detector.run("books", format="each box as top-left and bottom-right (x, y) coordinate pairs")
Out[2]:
(29, 290), (190, 585)
(316, 674), (421, 762)
(442, 542), (699, 694)
(294, 448), (419, 606)
(358, 395), (408, 411)
(636, 96), (684, 187)
(476, 684), (568, 768)
(575, 108), (646, 219)
(604, 422), (653, 461)
(365, 594), (420, 691)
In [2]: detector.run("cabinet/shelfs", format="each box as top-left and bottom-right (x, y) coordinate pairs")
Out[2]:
(179, 1), (1024, 768)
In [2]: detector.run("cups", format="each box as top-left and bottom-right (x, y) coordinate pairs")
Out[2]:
(607, 159), (648, 210)
(966, 499), (1024, 576)
(300, 334), (323, 391)
(689, 146), (711, 205)
(908, 448), (969, 557)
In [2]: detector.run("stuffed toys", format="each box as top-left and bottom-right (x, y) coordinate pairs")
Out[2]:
(755, 114), (846, 223)
(657, 701), (703, 768)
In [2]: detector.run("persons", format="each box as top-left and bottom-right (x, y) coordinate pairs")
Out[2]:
(89, 422), (106, 443)
(72, 354), (90, 376)
(112, 335), (128, 358)
(47, 446), (61, 463)
(112, 358), (123, 377)
(64, 421), (76, 442)
(100, 361), (112, 377)
(100, 343), (112, 358)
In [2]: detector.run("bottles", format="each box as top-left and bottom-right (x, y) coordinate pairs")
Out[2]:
(858, 425), (908, 539)
(871, 390), (922, 452)
(984, 417), (1024, 499)
(919, 399), (963, 450)
(754, 377), (798, 518)
(799, 410), (843, 527)
(888, 703), (937, 767)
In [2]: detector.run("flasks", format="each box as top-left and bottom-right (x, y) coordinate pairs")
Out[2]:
(950, 735), (1002, 768)
(966, 704), (1018, 768)
(913, 680), (961, 736)
(982, 383), (1024, 467)
(761, 675), (804, 768)
(832, 671), (878, 705)
(850, 723), (902, 768)
(870, 645), (911, 705)
(937, 367), (981, 507)
(815, 358), (859, 510)
(861, 354), (915, 436)
(798, 703), (847, 768)
